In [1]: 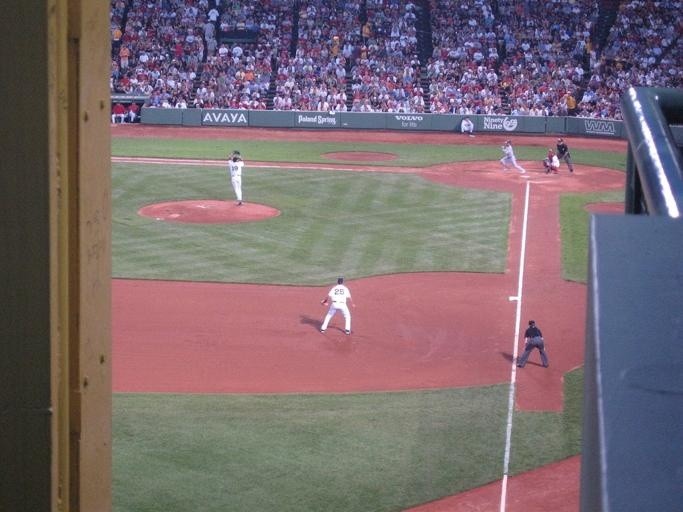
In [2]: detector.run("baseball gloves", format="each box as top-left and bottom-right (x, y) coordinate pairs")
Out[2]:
(320, 300), (328, 306)
(234, 150), (240, 159)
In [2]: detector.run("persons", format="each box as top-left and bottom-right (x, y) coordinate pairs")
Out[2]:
(542, 149), (560, 175)
(498, 139), (526, 174)
(108, 0), (681, 127)
(316, 277), (356, 336)
(556, 137), (573, 172)
(460, 117), (473, 135)
(513, 319), (549, 369)
(226, 150), (245, 206)
